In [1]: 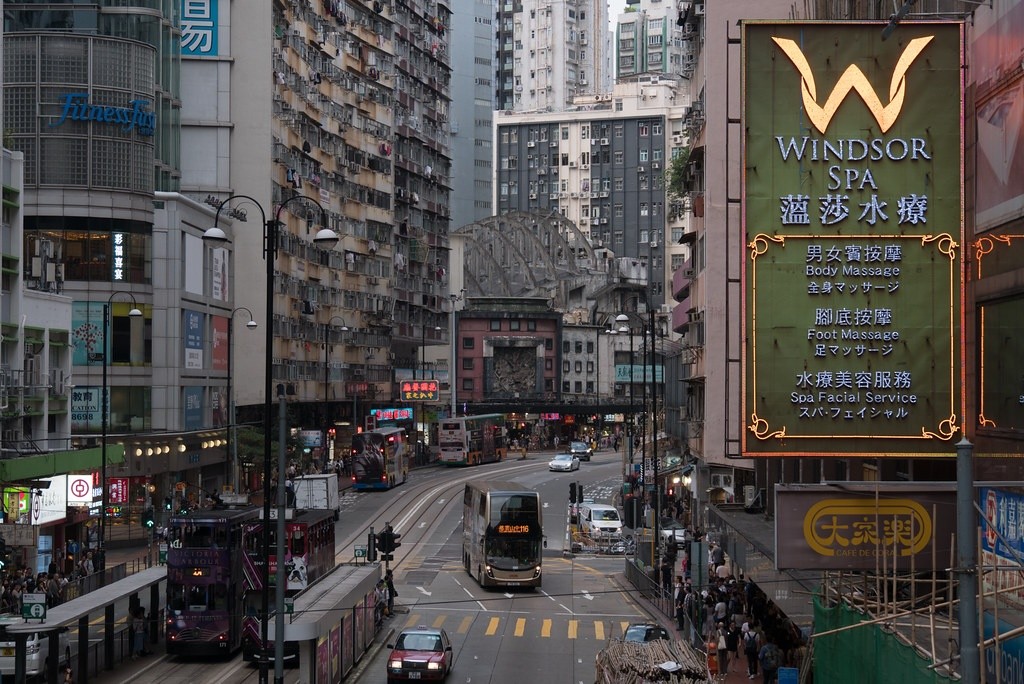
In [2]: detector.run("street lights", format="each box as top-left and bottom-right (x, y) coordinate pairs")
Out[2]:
(99, 290), (143, 588)
(615, 296), (662, 594)
(226, 306), (258, 486)
(421, 318), (444, 466)
(605, 313), (647, 517)
(199, 195), (340, 684)
(325, 315), (349, 472)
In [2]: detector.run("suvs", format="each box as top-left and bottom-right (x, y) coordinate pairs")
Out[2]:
(567, 440), (590, 462)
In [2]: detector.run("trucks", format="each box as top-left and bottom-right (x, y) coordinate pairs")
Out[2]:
(294, 473), (341, 523)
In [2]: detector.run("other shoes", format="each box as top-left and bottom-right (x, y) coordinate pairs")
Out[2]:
(676, 628), (683, 631)
(750, 675), (754, 679)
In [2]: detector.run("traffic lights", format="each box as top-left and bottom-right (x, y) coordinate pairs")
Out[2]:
(668, 487), (674, 496)
(376, 533), (386, 553)
(166, 496), (172, 510)
(568, 483), (576, 503)
(146, 509), (155, 529)
(385, 530), (401, 554)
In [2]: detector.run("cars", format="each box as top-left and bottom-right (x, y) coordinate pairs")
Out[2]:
(623, 623), (670, 644)
(387, 625), (454, 684)
(569, 498), (597, 524)
(636, 516), (687, 549)
(548, 453), (580, 473)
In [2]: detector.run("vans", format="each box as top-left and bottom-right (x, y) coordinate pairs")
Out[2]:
(580, 504), (624, 542)
(0, 611), (72, 684)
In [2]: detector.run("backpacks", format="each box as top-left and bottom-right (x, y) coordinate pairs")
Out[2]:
(746, 632), (757, 655)
(764, 645), (778, 669)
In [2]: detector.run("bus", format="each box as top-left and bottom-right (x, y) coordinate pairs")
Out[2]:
(350, 426), (408, 491)
(462, 480), (548, 591)
(165, 505), (340, 661)
(436, 413), (507, 467)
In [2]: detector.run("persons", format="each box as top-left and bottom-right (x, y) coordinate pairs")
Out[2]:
(661, 529), (801, 683)
(158, 489), (223, 564)
(0, 552), (94, 614)
(261, 455), (352, 503)
(507, 428), (640, 452)
(192, 526), (212, 548)
(374, 569), (396, 625)
(127, 607), (154, 662)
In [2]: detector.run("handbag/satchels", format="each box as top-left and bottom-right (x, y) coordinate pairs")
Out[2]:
(718, 630), (726, 649)
